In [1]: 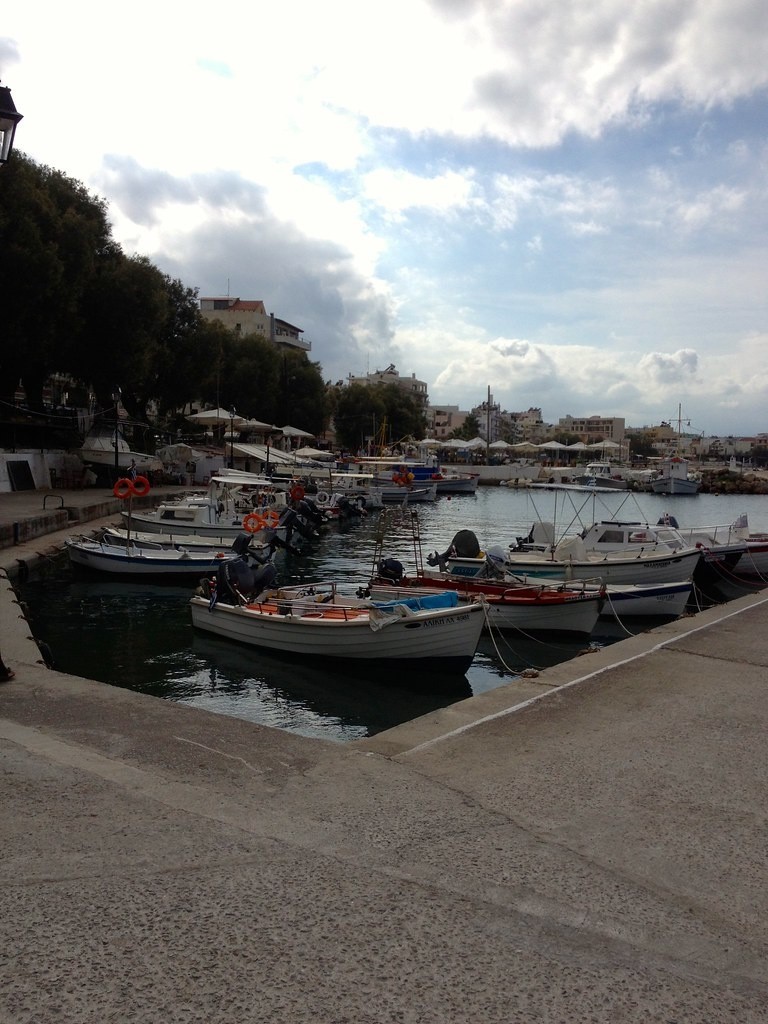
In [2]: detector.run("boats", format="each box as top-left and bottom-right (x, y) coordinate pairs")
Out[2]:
(356, 467), (768, 642)
(75, 435), (163, 478)
(573, 441), (628, 489)
(189, 532), (490, 676)
(65, 417), (480, 590)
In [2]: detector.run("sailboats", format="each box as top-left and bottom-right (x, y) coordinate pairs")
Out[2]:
(647, 403), (704, 495)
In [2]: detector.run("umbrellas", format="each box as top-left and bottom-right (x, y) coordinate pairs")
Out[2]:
(565, 442), (587, 451)
(183, 408), (242, 424)
(535, 441), (566, 450)
(489, 440), (510, 450)
(238, 418), (272, 432)
(508, 442), (543, 451)
(587, 440), (622, 450)
(441, 439), (473, 448)
(281, 425), (315, 439)
(421, 439), (441, 444)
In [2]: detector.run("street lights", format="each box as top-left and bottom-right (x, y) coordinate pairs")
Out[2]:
(228, 404), (237, 469)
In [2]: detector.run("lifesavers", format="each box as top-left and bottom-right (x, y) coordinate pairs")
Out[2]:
(356, 496), (366, 507)
(292, 486), (304, 499)
(262, 511), (279, 528)
(316, 492), (329, 504)
(113, 475), (150, 499)
(243, 514), (262, 533)
